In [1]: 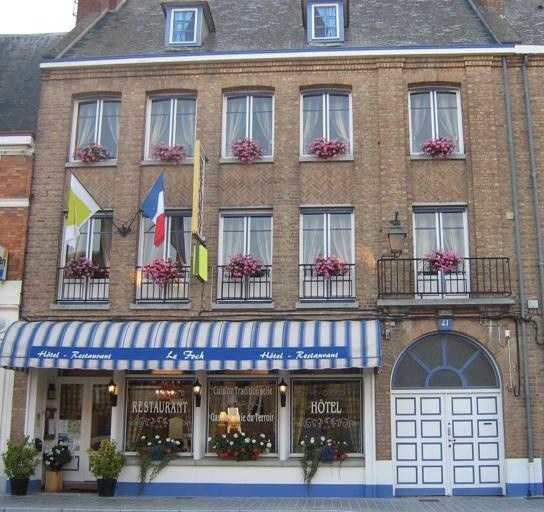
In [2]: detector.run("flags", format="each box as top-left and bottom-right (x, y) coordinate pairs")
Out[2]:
(140, 174), (166, 247)
(65, 174), (100, 248)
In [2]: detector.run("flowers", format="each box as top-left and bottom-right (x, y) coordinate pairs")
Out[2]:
(132, 433), (185, 497)
(208, 430), (271, 462)
(41, 441), (75, 470)
(297, 431), (350, 496)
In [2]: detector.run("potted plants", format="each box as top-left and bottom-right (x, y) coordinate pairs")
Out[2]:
(85, 438), (129, 496)
(0, 432), (40, 495)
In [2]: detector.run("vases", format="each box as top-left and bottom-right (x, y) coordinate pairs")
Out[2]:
(45, 470), (63, 492)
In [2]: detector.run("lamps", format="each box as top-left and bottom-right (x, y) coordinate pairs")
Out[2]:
(192, 377), (201, 407)
(388, 211), (406, 258)
(106, 377), (116, 406)
(277, 376), (287, 406)
